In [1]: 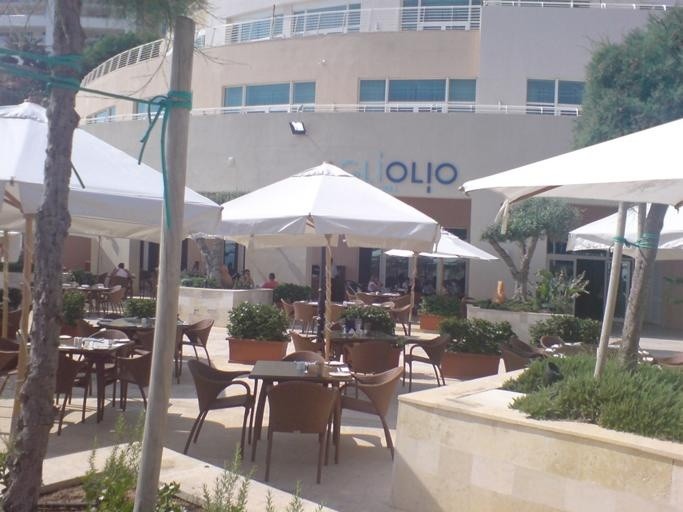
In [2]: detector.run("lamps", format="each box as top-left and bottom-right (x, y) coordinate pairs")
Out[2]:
(288, 120), (306, 135)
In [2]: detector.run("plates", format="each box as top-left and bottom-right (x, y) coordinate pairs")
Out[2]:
(324, 359), (352, 378)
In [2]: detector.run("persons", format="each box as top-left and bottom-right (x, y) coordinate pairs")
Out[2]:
(232, 269), (254, 290)
(112, 262), (135, 278)
(343, 275), (460, 299)
(148, 265), (158, 298)
(262, 273), (278, 288)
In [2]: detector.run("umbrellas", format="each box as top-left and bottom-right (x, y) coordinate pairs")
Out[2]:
(566, 206), (682, 260)
(383, 226), (500, 295)
(460, 117), (683, 206)
(0, 95), (223, 444)
(219, 162), (441, 364)
